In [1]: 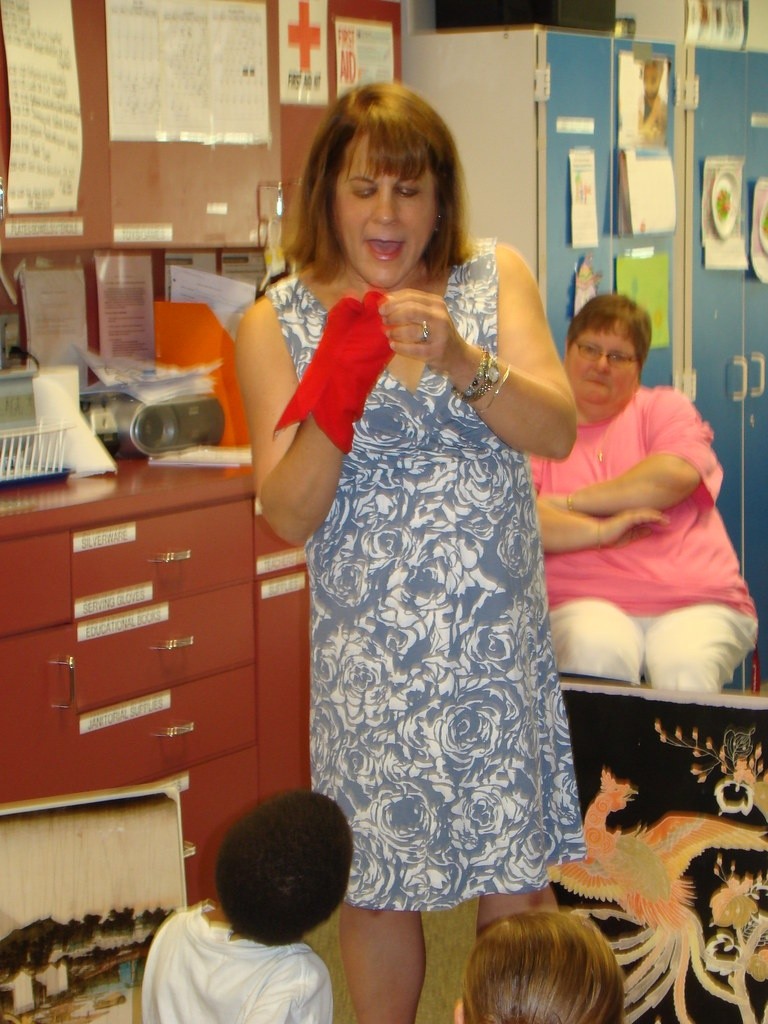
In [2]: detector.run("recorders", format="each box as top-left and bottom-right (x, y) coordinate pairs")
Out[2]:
(103, 394), (225, 458)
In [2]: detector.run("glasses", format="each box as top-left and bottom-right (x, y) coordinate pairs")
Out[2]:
(572, 337), (639, 372)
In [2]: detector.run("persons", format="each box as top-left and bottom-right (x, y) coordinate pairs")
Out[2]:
(140, 788), (353, 1024)
(526, 292), (757, 694)
(237, 83), (578, 1024)
(453, 910), (625, 1024)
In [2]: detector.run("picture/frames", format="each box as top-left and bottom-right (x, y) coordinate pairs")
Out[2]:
(555, 679), (768, 1024)
(0, 785), (187, 1024)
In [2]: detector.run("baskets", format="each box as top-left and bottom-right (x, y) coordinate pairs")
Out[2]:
(0, 418), (79, 488)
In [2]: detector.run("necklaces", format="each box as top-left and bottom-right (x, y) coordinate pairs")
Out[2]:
(580, 390), (643, 461)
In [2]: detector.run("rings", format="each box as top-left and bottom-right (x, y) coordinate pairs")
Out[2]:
(422, 319), (429, 341)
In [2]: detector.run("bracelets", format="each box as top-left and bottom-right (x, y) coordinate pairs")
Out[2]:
(597, 519), (601, 551)
(449, 343), (513, 412)
(567, 494), (572, 511)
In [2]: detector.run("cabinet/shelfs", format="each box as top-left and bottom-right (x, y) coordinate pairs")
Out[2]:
(410, 25), (768, 703)
(0, 0), (409, 247)
(0, 451), (337, 910)
